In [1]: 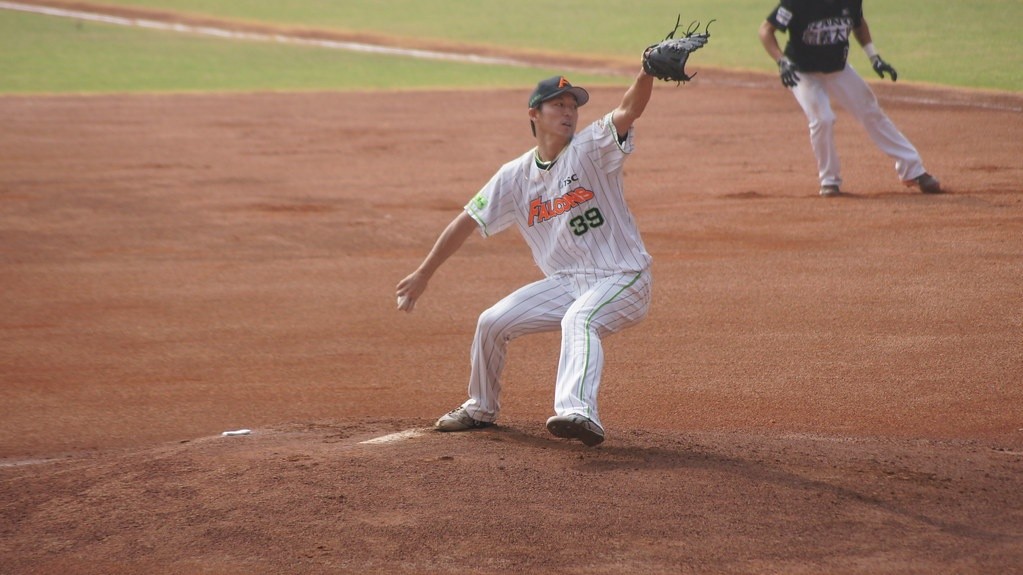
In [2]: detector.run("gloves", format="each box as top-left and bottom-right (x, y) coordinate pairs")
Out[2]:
(870, 54), (897, 81)
(777, 55), (800, 87)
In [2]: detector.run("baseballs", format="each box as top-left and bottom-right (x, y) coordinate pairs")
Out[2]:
(397, 295), (418, 310)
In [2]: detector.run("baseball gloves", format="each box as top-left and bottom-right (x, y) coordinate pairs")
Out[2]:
(642, 33), (710, 82)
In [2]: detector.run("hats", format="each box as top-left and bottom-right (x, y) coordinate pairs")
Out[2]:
(528, 76), (588, 108)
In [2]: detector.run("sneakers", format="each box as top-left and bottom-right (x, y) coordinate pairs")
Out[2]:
(818, 185), (839, 196)
(434, 406), (492, 431)
(903, 173), (940, 192)
(546, 413), (605, 447)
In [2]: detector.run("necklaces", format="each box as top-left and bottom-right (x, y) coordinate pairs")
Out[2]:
(522, 146), (555, 182)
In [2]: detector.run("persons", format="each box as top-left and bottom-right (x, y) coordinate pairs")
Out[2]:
(397, 15), (716, 446)
(758, 0), (944, 196)
